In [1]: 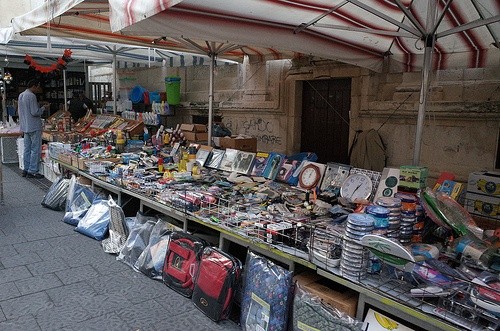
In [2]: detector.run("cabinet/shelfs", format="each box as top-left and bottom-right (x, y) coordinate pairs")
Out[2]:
(6, 73), (84, 114)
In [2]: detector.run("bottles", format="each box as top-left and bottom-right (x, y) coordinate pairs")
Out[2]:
(122, 100), (166, 126)
(449, 238), (494, 267)
(75, 129), (196, 172)
(362, 205), (391, 274)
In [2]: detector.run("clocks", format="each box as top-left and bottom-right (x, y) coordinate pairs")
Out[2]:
(340, 173), (373, 200)
(298, 164), (321, 190)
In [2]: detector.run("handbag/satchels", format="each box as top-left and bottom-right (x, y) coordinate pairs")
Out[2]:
(101, 194), (129, 253)
(74, 199), (111, 241)
(117, 213), (156, 272)
(62, 175), (97, 226)
(41, 174), (71, 212)
(134, 218), (178, 280)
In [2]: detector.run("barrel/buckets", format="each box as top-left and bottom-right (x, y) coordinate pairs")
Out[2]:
(149, 91), (160, 104)
(165, 76), (181, 106)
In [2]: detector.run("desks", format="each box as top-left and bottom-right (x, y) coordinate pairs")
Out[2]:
(49, 157), (464, 331)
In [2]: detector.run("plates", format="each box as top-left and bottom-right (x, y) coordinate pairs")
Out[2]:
(362, 234), (416, 271)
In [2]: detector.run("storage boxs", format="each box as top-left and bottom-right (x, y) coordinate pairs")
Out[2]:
(39, 103), (146, 185)
(180, 124), (500, 229)
(291, 272), (358, 318)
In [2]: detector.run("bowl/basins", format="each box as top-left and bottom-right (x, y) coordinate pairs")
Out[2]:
(144, 91), (166, 104)
(130, 86), (146, 103)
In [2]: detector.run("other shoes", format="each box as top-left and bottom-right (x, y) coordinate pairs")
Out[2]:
(26, 173), (44, 179)
(22, 170), (27, 177)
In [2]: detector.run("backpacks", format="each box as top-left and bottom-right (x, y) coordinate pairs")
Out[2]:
(192, 246), (242, 323)
(163, 231), (208, 298)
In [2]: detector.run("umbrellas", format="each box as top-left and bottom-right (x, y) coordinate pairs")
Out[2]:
(0, 1), (500, 167)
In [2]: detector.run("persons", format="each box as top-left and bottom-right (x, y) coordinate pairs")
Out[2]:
(78, 93), (97, 114)
(69, 90), (86, 121)
(18, 79), (50, 179)
